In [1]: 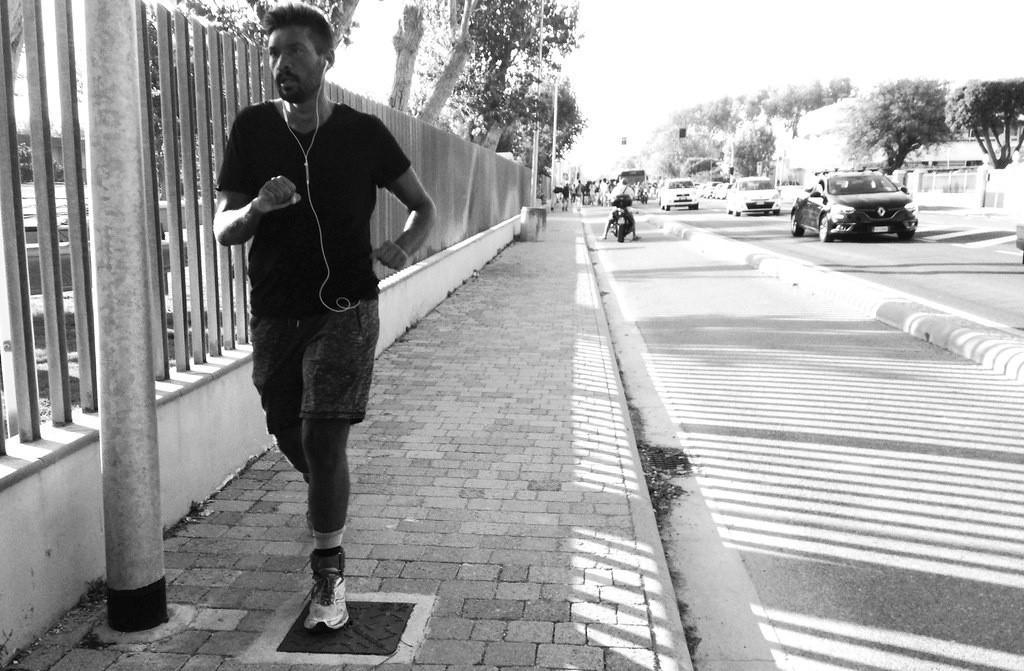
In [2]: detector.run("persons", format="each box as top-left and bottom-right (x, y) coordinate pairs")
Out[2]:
(560, 177), (646, 240)
(211, 2), (438, 634)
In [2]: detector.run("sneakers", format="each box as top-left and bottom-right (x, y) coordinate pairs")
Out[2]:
(304, 567), (349, 631)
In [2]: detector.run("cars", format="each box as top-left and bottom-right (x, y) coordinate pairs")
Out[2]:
(791, 167), (918, 243)
(643, 180), (732, 200)
(726, 177), (781, 216)
(658, 178), (700, 211)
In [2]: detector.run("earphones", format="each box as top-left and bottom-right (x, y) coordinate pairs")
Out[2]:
(321, 59), (329, 75)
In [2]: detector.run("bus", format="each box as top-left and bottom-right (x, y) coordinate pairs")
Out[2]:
(617, 169), (648, 201)
